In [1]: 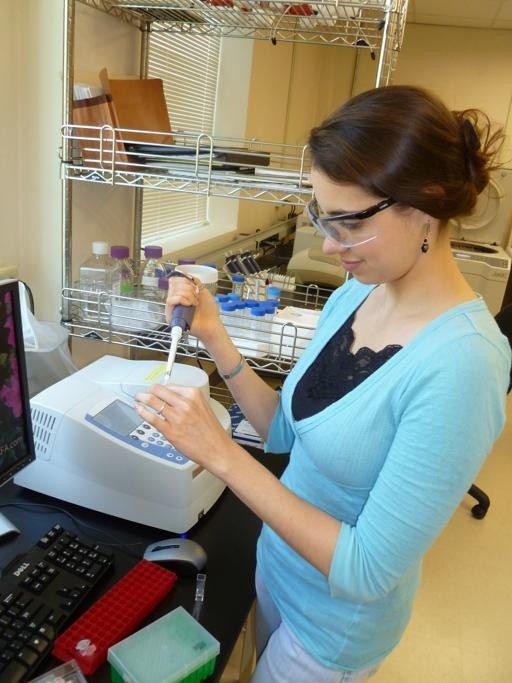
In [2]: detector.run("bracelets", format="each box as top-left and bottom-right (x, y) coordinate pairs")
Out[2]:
(220, 353), (245, 380)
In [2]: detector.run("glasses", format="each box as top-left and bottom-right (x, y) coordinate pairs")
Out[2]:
(306, 199), (397, 247)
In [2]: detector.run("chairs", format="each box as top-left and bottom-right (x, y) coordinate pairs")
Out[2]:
(469, 303), (512, 519)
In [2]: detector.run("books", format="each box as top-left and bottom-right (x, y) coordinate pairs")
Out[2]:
(139, 152), (313, 194)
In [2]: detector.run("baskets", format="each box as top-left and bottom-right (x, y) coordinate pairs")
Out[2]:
(76, 0), (408, 49)
(60, 125), (316, 206)
(62, 260), (334, 376)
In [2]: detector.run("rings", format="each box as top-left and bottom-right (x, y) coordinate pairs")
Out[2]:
(157, 403), (166, 415)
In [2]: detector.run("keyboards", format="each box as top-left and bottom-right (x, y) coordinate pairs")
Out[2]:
(0, 522), (116, 683)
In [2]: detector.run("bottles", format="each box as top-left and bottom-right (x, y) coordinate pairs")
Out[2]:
(77, 240), (281, 359)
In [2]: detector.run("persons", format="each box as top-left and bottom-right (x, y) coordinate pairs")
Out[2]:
(132, 85), (511, 683)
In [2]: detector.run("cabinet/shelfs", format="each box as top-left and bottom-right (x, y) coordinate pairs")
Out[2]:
(58, 0), (410, 451)
(0, 444), (290, 682)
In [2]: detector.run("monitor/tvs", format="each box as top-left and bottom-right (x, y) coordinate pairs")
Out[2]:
(0, 277), (37, 537)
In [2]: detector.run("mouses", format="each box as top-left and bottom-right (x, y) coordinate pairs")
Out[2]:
(143, 537), (208, 572)
